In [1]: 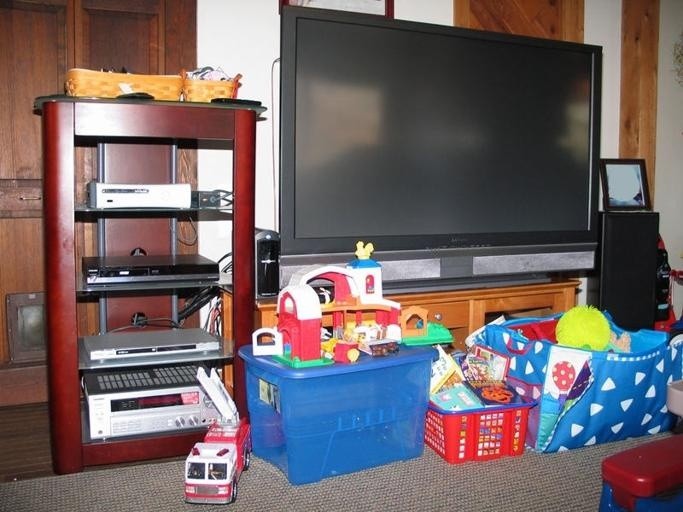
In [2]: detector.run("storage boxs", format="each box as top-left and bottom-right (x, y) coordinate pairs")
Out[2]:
(238, 344), (438, 485)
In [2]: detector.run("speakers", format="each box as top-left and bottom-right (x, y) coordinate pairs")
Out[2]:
(251, 226), (284, 303)
(592, 211), (661, 333)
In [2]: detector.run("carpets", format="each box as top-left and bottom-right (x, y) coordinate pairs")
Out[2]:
(1, 434), (683, 512)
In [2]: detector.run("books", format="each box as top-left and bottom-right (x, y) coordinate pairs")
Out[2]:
(430, 344), (525, 411)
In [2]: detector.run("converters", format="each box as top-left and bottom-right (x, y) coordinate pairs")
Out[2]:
(190, 189), (224, 209)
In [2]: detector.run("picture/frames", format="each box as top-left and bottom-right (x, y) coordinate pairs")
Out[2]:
(598, 157), (652, 210)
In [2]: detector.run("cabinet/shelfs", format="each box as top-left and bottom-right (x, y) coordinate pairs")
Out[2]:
(32, 94), (267, 477)
(0, 0), (195, 410)
(252, 281), (583, 353)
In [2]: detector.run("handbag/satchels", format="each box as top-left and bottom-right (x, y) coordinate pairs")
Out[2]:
(486, 310), (683, 452)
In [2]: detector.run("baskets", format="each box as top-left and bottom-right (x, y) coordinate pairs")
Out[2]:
(66, 68), (182, 101)
(424, 383), (539, 464)
(180, 69), (242, 104)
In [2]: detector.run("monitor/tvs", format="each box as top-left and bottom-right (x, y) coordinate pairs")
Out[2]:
(278, 0), (604, 297)
